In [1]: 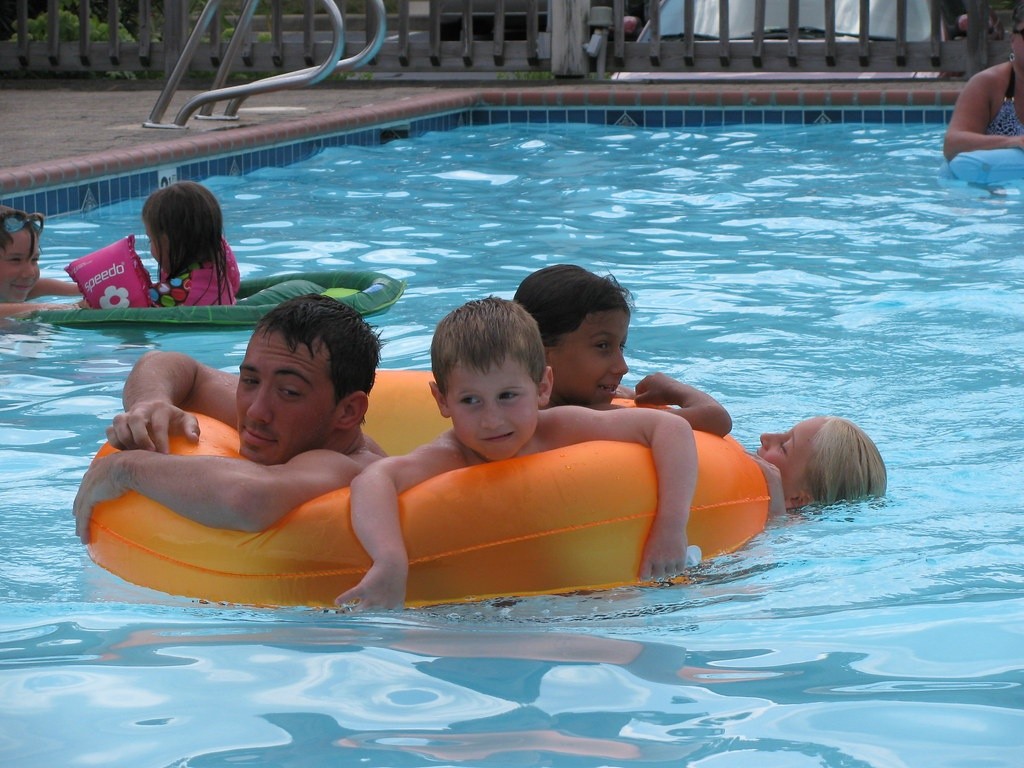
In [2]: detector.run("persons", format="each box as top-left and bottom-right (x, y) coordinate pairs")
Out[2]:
(517, 265), (733, 440)
(333, 292), (699, 615)
(755, 415), (887, 511)
(82, 180), (241, 309)
(72, 292), (388, 546)
(0, 206), (82, 333)
(943, 0), (1024, 160)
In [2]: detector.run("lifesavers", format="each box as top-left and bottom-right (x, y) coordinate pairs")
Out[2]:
(63, 236), (153, 309)
(15, 267), (410, 332)
(82, 366), (774, 609)
(949, 148), (1024, 184)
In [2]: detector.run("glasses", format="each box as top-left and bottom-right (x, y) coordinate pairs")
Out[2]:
(3, 213), (41, 234)
